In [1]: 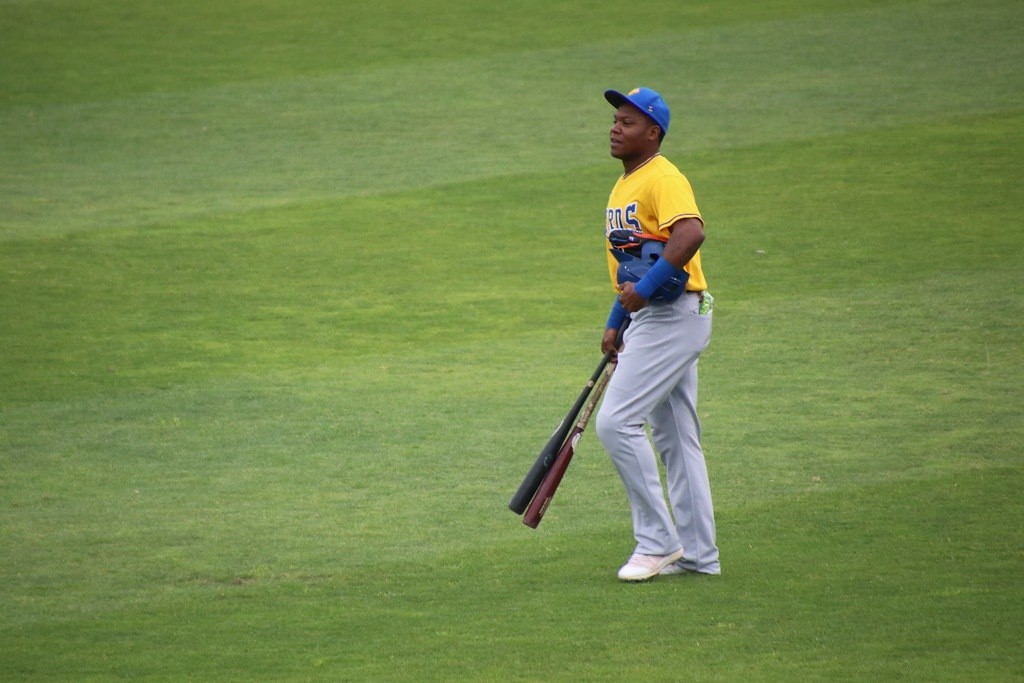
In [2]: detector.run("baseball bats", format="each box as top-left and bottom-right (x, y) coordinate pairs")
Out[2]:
(508, 311), (632, 515)
(522, 356), (616, 530)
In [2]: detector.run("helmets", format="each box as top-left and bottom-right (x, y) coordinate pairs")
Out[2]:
(609, 242), (689, 303)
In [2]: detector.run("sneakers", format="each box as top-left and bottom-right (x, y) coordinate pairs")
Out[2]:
(658, 564), (693, 575)
(617, 547), (685, 580)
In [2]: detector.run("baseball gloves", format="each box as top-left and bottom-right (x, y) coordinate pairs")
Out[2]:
(609, 229), (668, 256)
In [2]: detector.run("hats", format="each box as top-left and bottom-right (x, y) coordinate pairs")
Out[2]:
(604, 86), (670, 134)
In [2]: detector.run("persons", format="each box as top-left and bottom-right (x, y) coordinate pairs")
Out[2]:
(601, 87), (719, 581)
